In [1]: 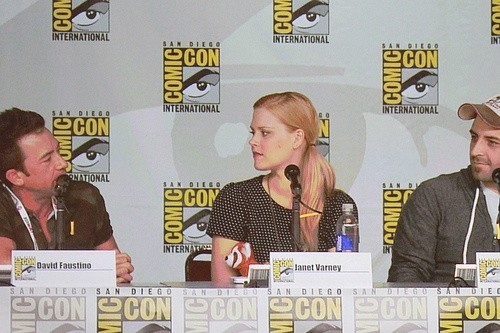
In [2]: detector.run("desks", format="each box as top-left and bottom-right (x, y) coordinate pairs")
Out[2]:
(0, 282), (500, 333)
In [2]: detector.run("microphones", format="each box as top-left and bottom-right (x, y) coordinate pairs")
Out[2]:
(285, 165), (302, 193)
(491, 167), (500, 186)
(54, 175), (72, 197)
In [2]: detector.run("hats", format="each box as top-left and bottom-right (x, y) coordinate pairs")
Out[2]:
(457, 95), (500, 129)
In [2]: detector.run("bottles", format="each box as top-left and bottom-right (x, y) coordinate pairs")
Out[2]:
(336, 203), (358, 252)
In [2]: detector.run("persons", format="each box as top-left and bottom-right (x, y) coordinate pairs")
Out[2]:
(0, 107), (135, 285)
(206, 90), (360, 282)
(387, 94), (500, 283)
